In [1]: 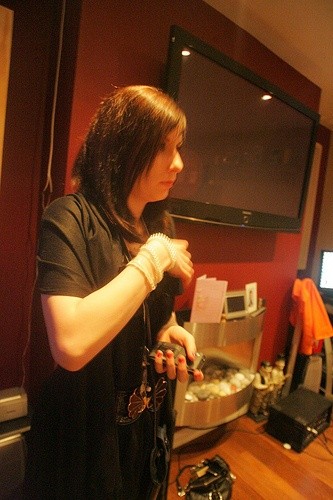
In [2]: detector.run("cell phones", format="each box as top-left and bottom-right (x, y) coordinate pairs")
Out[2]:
(149, 341), (206, 374)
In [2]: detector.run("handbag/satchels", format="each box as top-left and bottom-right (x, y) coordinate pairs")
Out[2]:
(175, 455), (233, 500)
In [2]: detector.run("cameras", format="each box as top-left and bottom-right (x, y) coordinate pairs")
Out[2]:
(121, 254), (182, 312)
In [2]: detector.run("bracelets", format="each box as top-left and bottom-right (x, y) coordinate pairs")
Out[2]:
(124, 232), (178, 292)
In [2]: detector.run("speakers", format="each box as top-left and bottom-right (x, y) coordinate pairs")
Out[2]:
(267, 387), (332, 453)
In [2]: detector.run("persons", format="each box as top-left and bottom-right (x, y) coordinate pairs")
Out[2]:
(19, 84), (205, 500)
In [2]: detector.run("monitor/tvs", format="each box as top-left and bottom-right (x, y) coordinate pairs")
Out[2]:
(162, 23), (322, 231)
(318, 249), (333, 289)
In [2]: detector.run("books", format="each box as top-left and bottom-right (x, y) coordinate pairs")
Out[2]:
(190, 274), (228, 324)
